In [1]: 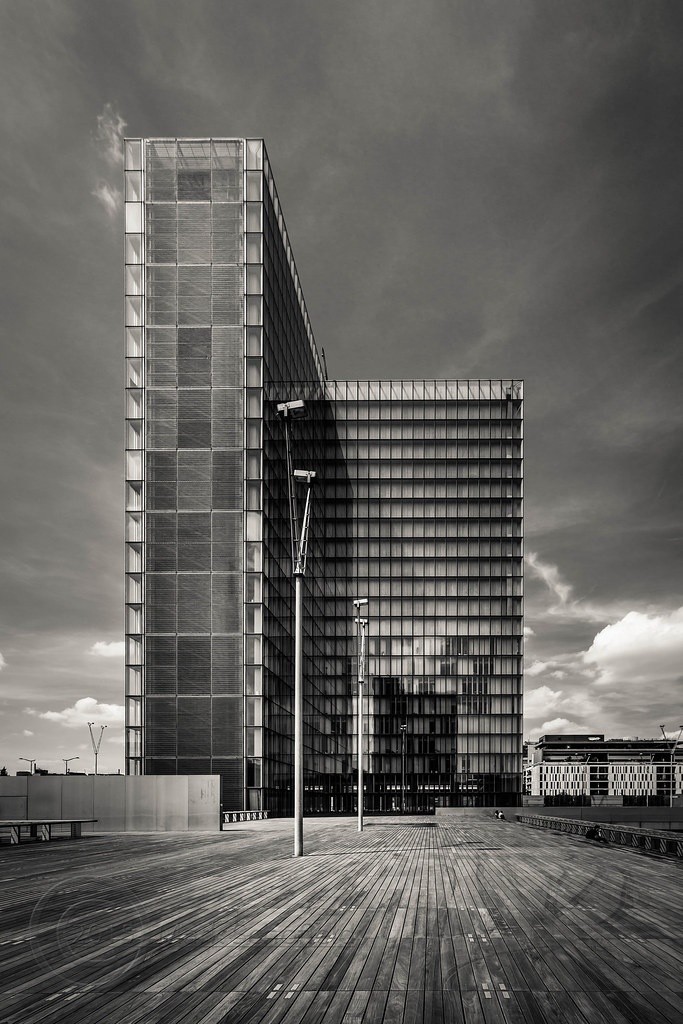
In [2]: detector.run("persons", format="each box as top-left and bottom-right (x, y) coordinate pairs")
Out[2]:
(592, 825), (610, 844)
(494, 810), (504, 818)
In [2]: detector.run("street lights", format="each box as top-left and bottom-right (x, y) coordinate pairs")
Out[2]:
(352, 597), (369, 833)
(62, 756), (80, 776)
(659, 725), (683, 808)
(19, 757), (36, 774)
(276, 397), (316, 856)
(88, 722), (107, 776)
(400, 724), (408, 814)
